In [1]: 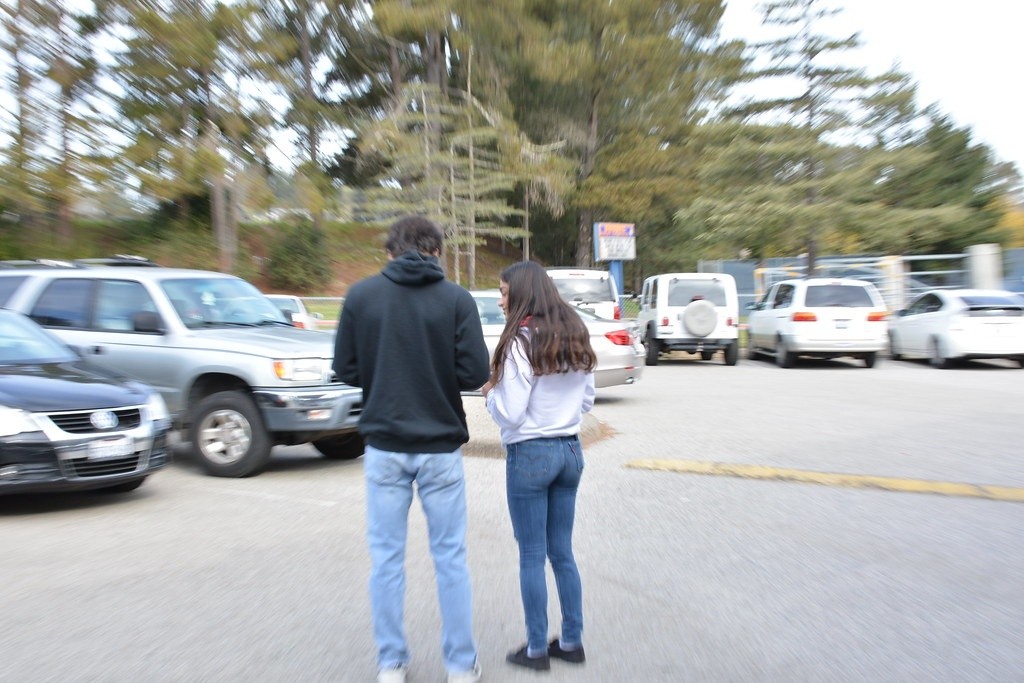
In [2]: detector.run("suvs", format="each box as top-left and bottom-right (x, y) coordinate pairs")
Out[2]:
(0, 256), (370, 479)
(636, 272), (739, 366)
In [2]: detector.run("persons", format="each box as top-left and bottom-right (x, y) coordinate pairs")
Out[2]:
(332, 216), (490, 683)
(479, 259), (598, 673)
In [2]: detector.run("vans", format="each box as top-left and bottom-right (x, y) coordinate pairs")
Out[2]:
(540, 268), (621, 320)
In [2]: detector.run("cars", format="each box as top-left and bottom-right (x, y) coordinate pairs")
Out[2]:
(886, 290), (1023, 367)
(264, 292), (324, 332)
(745, 279), (887, 369)
(0, 308), (172, 500)
(458, 289), (646, 392)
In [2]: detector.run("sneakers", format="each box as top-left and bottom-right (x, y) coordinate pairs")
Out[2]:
(548, 639), (587, 666)
(447, 658), (482, 681)
(376, 668), (406, 681)
(506, 643), (551, 673)
